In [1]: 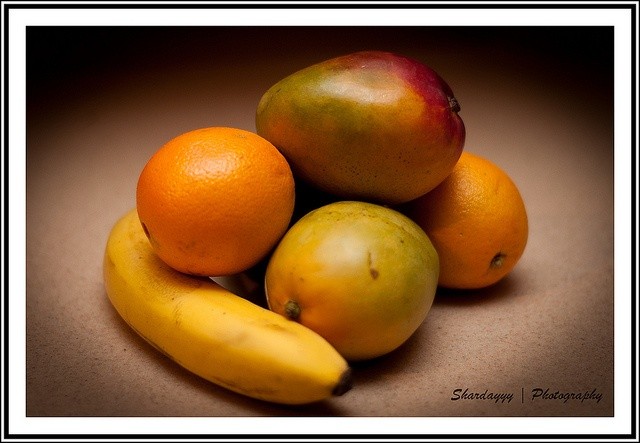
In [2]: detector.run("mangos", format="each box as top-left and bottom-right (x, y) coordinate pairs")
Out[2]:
(254, 50), (466, 200)
(264, 202), (440, 360)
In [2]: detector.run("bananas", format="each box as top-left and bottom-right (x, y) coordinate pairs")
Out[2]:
(103, 205), (355, 406)
